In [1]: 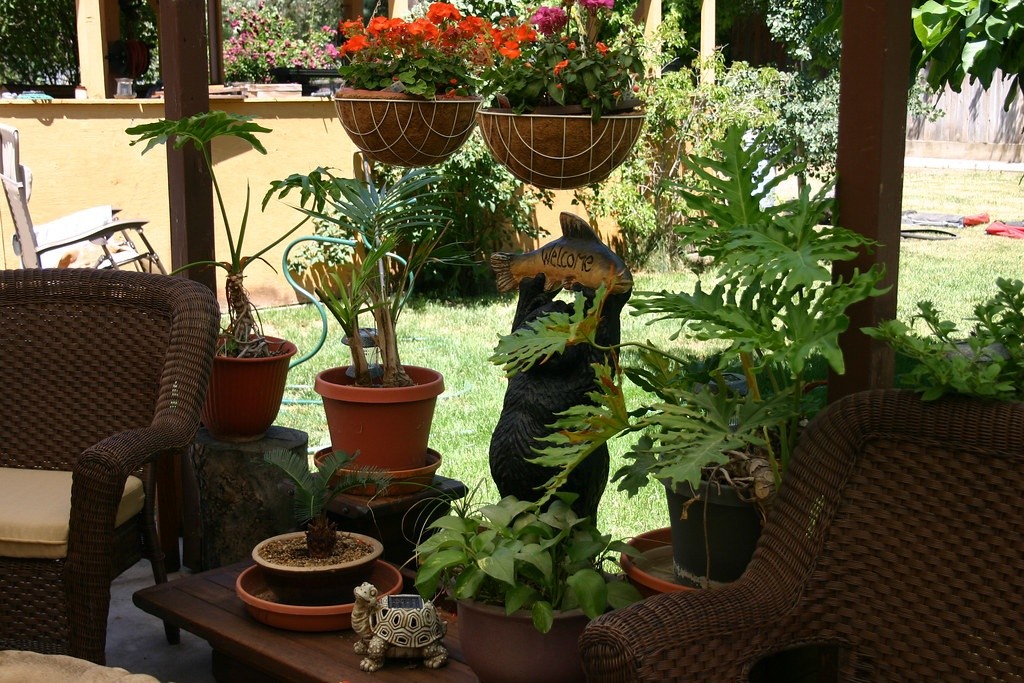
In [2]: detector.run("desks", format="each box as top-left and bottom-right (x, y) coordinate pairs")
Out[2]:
(133, 559), (479, 683)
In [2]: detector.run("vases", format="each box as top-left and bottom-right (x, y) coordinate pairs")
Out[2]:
(335, 87), (482, 166)
(475, 110), (648, 190)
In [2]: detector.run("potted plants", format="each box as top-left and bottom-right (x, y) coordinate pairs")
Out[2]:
(412, 495), (647, 683)
(125, 113), (297, 441)
(261, 164), (457, 498)
(235, 447), (403, 633)
(492, 121), (893, 595)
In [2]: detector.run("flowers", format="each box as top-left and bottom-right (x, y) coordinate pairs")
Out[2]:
(478, 0), (640, 108)
(340, 2), (538, 98)
(222, 0), (339, 78)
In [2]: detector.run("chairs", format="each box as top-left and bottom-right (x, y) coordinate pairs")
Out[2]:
(578, 388), (1024, 683)
(0, 125), (167, 276)
(0, 270), (220, 666)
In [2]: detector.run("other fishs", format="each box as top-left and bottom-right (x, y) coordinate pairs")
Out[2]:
(489, 211), (633, 295)
